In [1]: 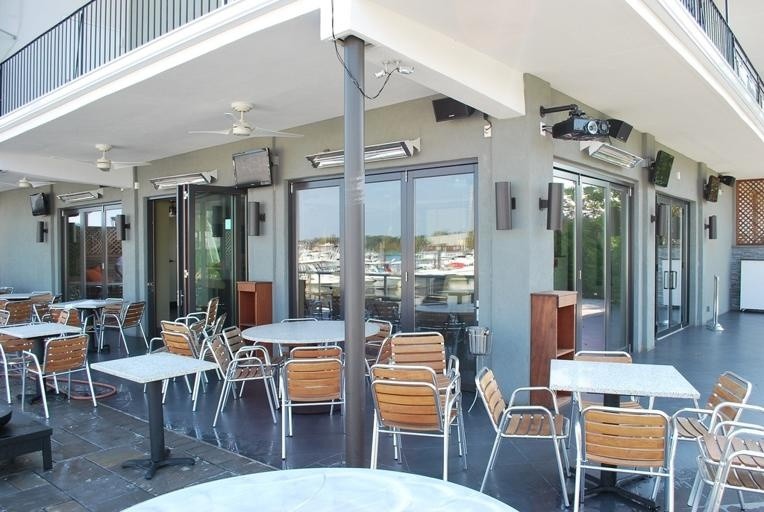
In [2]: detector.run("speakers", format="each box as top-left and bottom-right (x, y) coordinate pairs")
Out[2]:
(431, 97), (474, 122)
(606, 119), (633, 144)
(720, 175), (736, 187)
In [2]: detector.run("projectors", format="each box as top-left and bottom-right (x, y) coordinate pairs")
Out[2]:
(552, 116), (611, 143)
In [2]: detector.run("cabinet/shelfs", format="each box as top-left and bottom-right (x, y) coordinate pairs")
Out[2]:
(531, 289), (579, 410)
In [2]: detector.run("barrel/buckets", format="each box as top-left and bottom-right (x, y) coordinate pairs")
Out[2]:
(466, 326), (489, 355)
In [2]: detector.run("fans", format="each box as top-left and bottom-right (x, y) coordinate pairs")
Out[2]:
(187, 102), (304, 141)
(1, 178), (56, 189)
(65, 143), (150, 173)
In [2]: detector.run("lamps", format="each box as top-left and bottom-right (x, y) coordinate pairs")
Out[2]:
(150, 169), (217, 194)
(540, 183), (563, 230)
(705, 216), (717, 239)
(579, 139), (644, 170)
(56, 188), (104, 203)
(115, 215), (130, 240)
(247, 202), (266, 236)
(494, 182), (517, 230)
(303, 138), (420, 167)
(35, 221), (48, 242)
(651, 203), (671, 236)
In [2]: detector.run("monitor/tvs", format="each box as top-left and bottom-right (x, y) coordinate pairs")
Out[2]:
(231, 147), (273, 189)
(705, 174), (721, 203)
(650, 150), (675, 188)
(29, 191), (48, 216)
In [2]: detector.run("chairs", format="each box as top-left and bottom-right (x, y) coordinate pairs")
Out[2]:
(147, 294), (282, 430)
(367, 364), (461, 482)
(277, 344), (347, 462)
(574, 403), (679, 512)
(572, 350), (650, 424)
(688, 400), (763, 512)
(301, 285), (466, 378)
(390, 330), (468, 462)
(651, 369), (753, 501)
(0, 283), (151, 420)
(474, 364), (574, 509)
(277, 316), (324, 360)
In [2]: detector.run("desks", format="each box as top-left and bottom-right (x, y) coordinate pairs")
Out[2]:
(88, 351), (221, 482)
(548, 359), (700, 511)
(241, 321), (381, 420)
(1, 397), (54, 478)
(118, 468), (520, 512)
